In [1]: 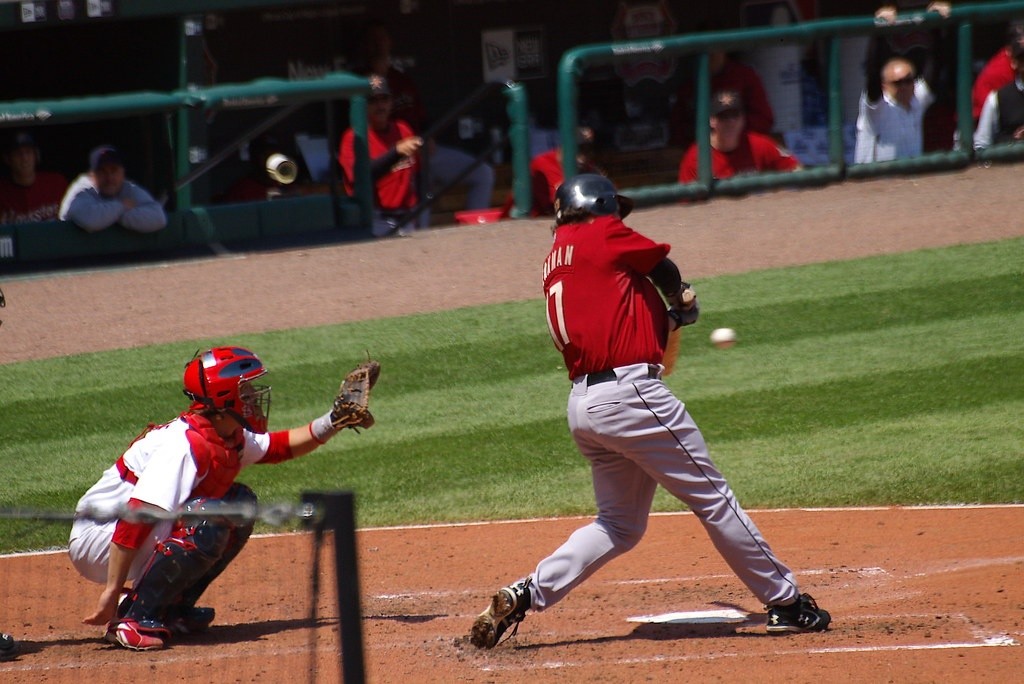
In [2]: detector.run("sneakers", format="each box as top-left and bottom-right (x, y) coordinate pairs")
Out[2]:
(765, 592), (830, 633)
(173, 607), (217, 634)
(104, 618), (171, 651)
(470, 575), (533, 649)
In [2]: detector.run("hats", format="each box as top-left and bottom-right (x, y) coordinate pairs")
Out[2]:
(362, 75), (389, 99)
(707, 88), (747, 120)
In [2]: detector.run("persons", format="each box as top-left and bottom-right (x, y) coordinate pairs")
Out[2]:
(69, 346), (380, 651)
(469, 170), (831, 650)
(0, 3), (1024, 234)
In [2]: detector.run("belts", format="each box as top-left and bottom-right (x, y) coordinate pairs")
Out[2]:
(584, 365), (660, 388)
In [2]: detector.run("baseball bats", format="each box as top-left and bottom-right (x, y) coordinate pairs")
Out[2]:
(662, 290), (693, 375)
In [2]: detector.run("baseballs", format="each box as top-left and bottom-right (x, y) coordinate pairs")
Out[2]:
(710, 327), (737, 348)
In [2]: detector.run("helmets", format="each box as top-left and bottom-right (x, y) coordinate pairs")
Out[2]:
(552, 175), (636, 222)
(183, 344), (268, 434)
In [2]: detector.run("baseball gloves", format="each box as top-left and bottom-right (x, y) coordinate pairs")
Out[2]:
(329, 360), (380, 429)
